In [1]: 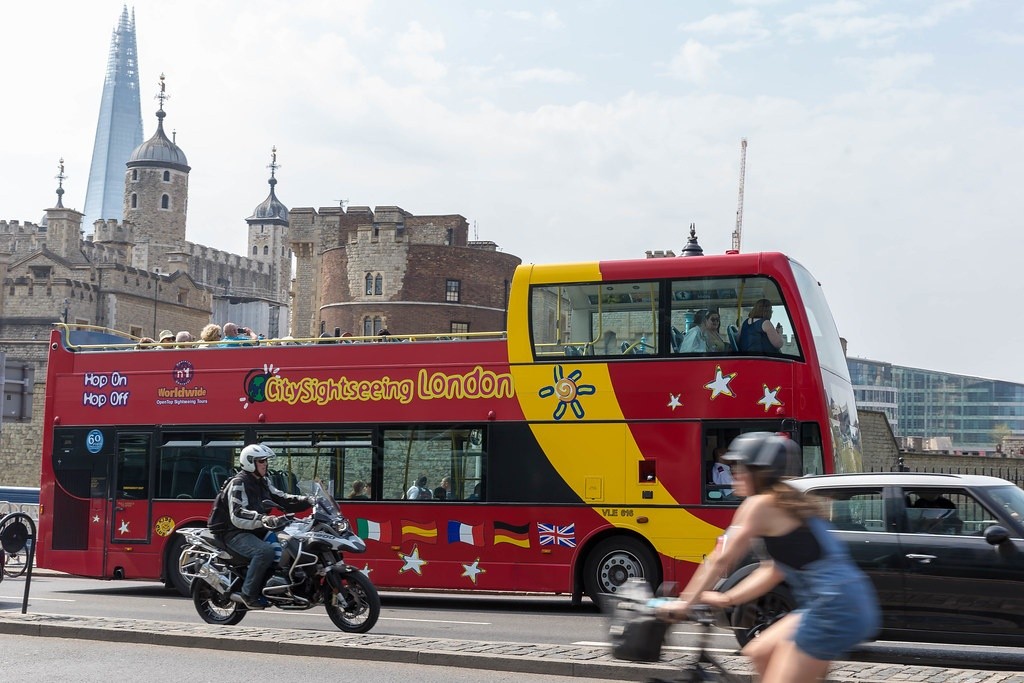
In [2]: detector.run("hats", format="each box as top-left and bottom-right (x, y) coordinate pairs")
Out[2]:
(159, 330), (174, 343)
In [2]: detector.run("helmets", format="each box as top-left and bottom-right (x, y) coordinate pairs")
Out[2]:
(239, 444), (276, 477)
(722, 431), (802, 477)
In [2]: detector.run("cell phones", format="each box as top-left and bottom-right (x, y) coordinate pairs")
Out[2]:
(237, 329), (243, 333)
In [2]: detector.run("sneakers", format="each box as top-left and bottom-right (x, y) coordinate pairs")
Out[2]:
(240, 591), (266, 610)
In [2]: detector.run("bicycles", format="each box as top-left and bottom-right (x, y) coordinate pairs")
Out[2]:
(597, 592), (878, 683)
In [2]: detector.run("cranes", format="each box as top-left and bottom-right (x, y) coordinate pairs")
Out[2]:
(732, 140), (748, 251)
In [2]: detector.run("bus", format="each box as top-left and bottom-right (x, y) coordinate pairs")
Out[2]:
(37, 224), (862, 618)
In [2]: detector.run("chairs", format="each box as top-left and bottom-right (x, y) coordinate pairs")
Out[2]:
(176, 465), (301, 496)
(562, 324), (739, 354)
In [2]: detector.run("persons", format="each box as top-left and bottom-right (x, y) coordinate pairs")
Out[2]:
(135, 323), (257, 349)
(712, 447), (739, 501)
(217, 444), (317, 609)
(258, 333), (266, 340)
(660, 432), (879, 683)
(401, 475), (433, 500)
(738, 299), (784, 353)
(680, 310), (725, 354)
(468, 483), (481, 500)
(348, 480), (371, 499)
(433, 476), (451, 500)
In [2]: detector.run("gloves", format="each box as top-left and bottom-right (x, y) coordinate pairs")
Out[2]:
(262, 515), (279, 528)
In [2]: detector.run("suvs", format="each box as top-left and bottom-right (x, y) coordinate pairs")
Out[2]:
(714, 472), (1024, 649)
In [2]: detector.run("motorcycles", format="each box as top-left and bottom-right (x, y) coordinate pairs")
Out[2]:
(175, 479), (380, 634)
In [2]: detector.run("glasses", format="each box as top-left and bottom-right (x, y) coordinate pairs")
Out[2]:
(259, 459), (268, 463)
(710, 316), (721, 322)
